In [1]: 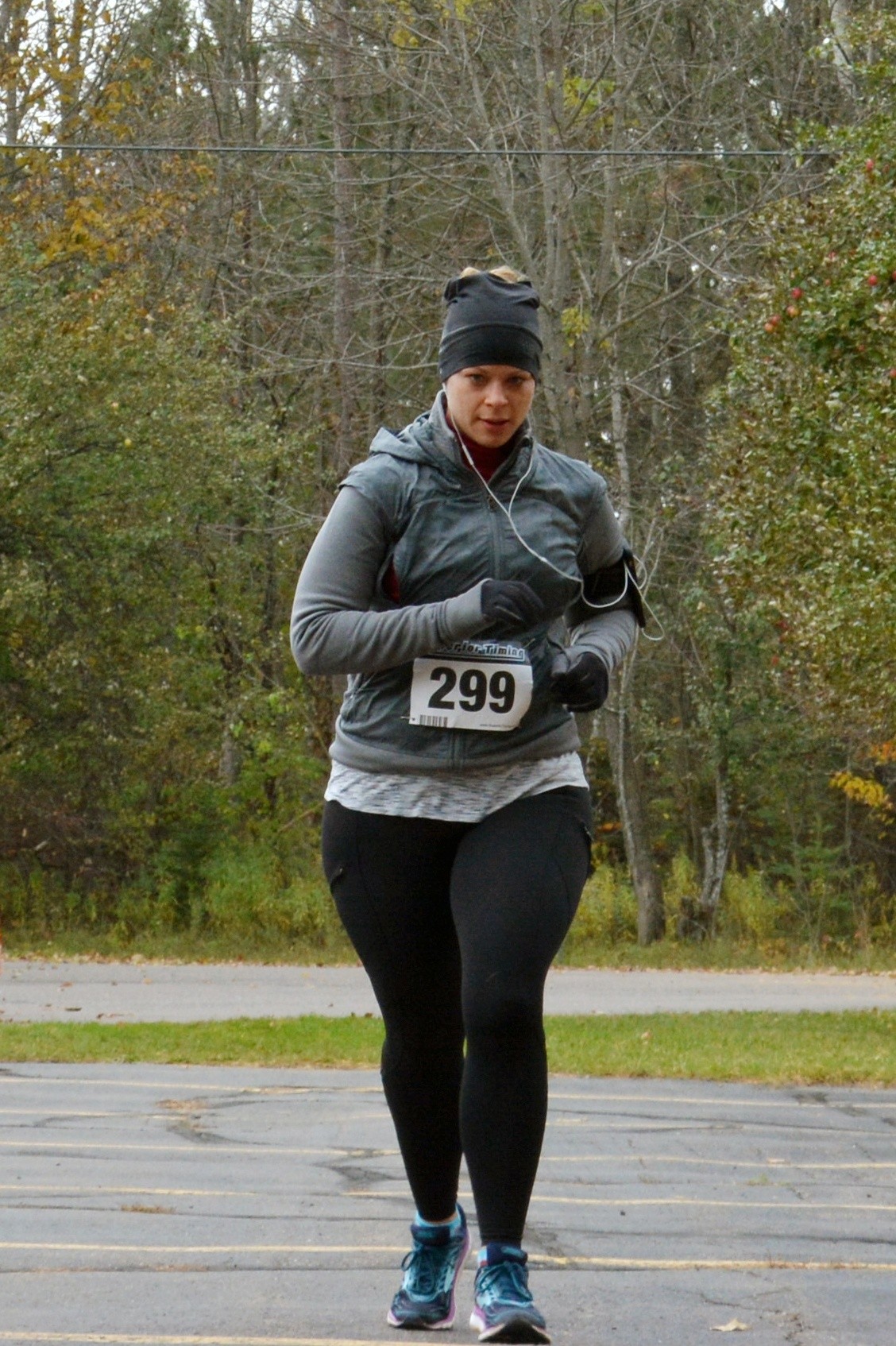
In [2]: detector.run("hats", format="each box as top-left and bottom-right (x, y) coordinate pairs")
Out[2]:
(439, 270), (544, 383)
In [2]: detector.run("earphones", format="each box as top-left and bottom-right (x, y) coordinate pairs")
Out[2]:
(442, 382), (446, 392)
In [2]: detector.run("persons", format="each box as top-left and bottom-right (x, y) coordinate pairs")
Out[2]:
(289, 266), (649, 1345)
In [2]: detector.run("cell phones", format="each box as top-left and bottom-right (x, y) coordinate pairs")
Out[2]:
(622, 537), (646, 629)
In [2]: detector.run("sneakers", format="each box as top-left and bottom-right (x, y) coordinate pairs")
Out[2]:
(469, 1241), (551, 1344)
(386, 1202), (471, 1329)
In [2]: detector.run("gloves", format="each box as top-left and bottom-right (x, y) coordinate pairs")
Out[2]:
(548, 652), (608, 713)
(481, 579), (541, 627)
